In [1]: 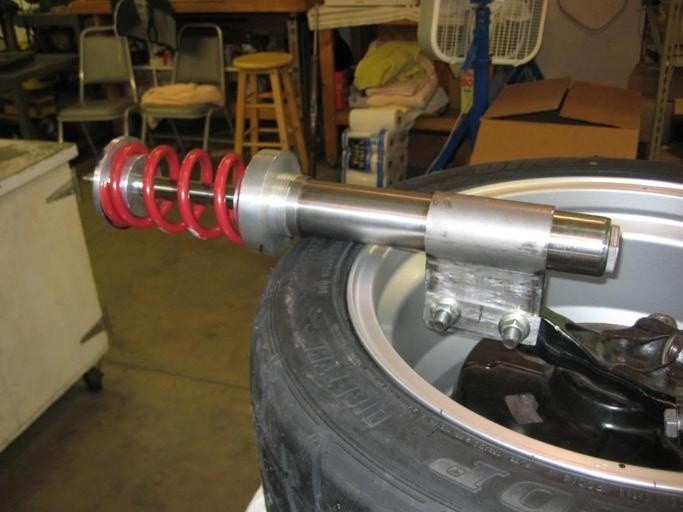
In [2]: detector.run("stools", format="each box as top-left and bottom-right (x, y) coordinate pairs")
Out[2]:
(229, 50), (308, 179)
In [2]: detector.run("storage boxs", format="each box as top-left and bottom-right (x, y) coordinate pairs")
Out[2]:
(467, 72), (643, 169)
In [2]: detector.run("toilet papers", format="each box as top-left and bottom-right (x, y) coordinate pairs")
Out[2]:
(339, 107), (414, 189)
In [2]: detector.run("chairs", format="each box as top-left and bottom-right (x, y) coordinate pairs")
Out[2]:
(54, 23), (234, 169)
